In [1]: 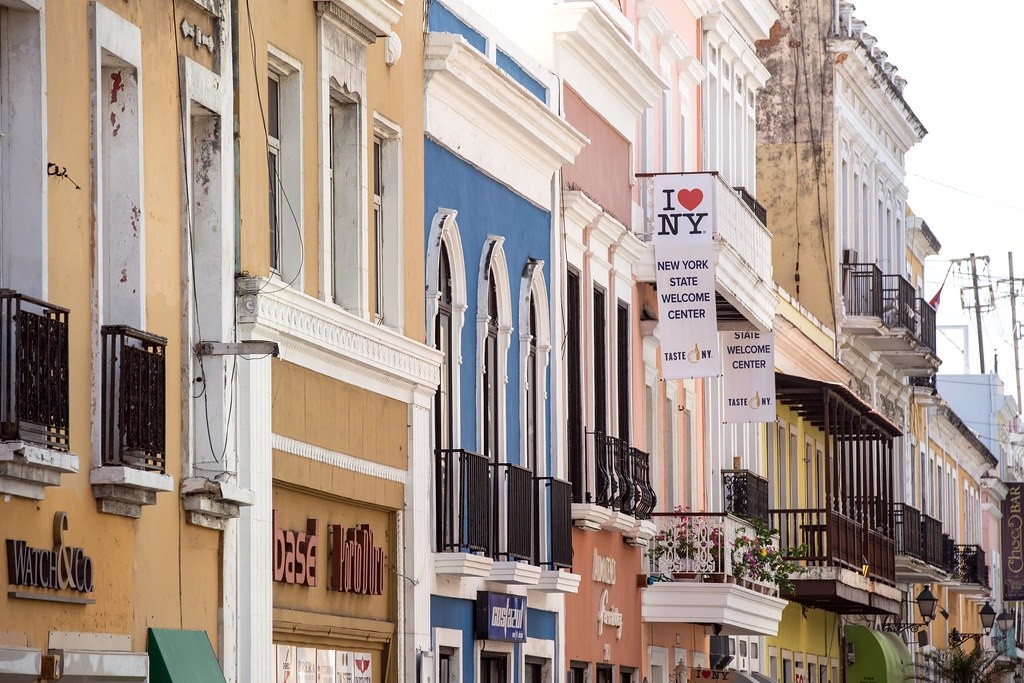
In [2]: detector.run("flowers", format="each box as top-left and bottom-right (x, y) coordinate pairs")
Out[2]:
(648, 504), (808, 595)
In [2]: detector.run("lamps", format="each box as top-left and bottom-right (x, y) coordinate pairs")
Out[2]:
(884, 584), (941, 634)
(947, 601), (998, 648)
(991, 607), (1015, 646)
